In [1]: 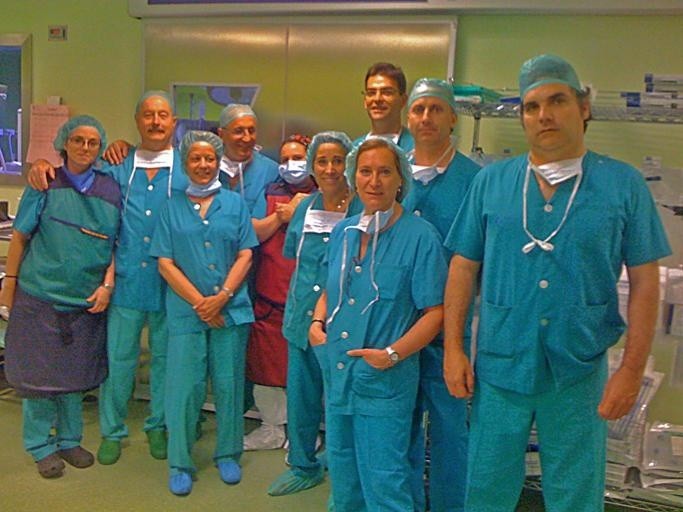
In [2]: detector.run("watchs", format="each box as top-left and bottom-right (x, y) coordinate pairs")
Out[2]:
(221, 287), (234, 297)
(101, 282), (113, 293)
(385, 347), (400, 365)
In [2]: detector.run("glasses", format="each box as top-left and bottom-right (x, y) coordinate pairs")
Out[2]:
(360, 86), (400, 98)
(67, 134), (101, 153)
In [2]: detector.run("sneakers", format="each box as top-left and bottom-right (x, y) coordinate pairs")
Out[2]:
(267, 469), (326, 497)
(286, 433), (321, 464)
(215, 460), (242, 484)
(35, 450), (67, 479)
(56, 444), (95, 469)
(242, 425), (286, 452)
(145, 429), (168, 460)
(168, 470), (193, 496)
(97, 438), (121, 466)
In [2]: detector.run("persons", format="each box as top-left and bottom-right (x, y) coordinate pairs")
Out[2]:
(307, 137), (446, 511)
(397, 79), (483, 511)
(268, 130), (359, 497)
(0, 115), (123, 476)
(28, 89), (191, 466)
(240, 133), (323, 467)
(213, 104), (284, 419)
(345, 60), (417, 169)
(150, 130), (260, 496)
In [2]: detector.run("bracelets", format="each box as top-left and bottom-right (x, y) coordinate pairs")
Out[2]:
(5, 275), (17, 278)
(311, 319), (324, 324)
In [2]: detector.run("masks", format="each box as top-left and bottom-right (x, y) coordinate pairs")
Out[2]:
(406, 139), (457, 186)
(325, 197), (396, 325)
(218, 153), (254, 194)
(522, 152), (587, 256)
(124, 145), (174, 213)
(278, 159), (311, 187)
(287, 192), (349, 328)
(184, 169), (221, 199)
